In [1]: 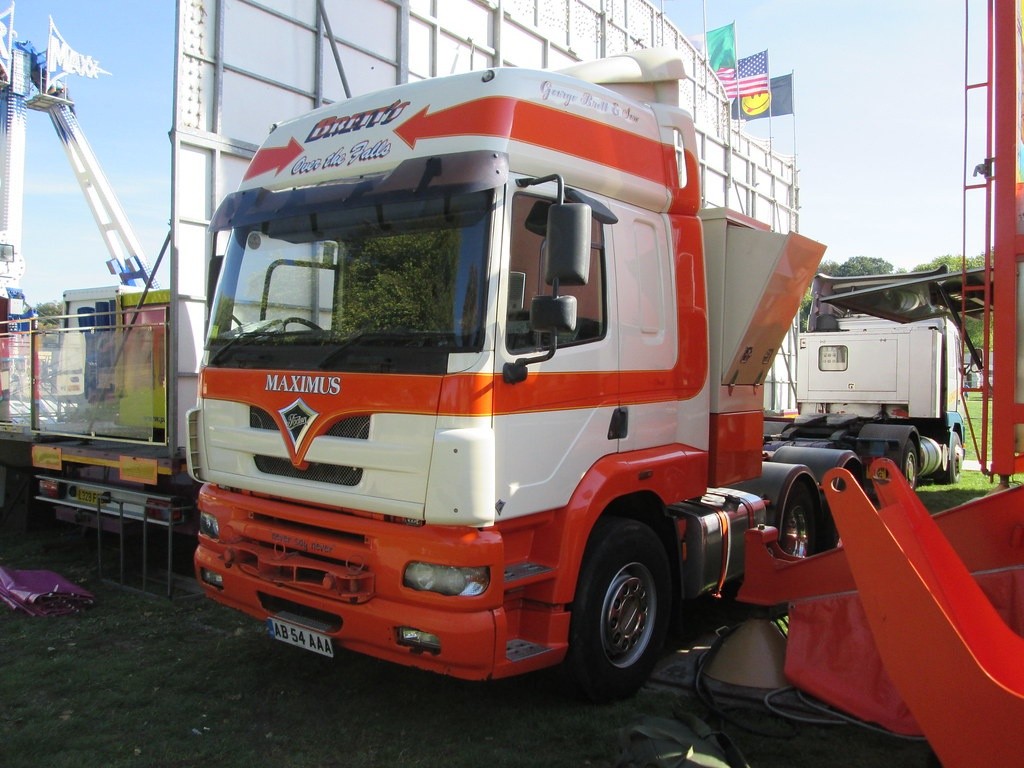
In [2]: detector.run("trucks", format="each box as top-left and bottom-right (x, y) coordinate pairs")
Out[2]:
(182, 42), (867, 702)
(763, 261), (986, 497)
(33, 429), (197, 543)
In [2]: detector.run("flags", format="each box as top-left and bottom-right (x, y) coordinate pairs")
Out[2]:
(716, 49), (769, 98)
(730, 73), (793, 122)
(686, 21), (736, 72)
